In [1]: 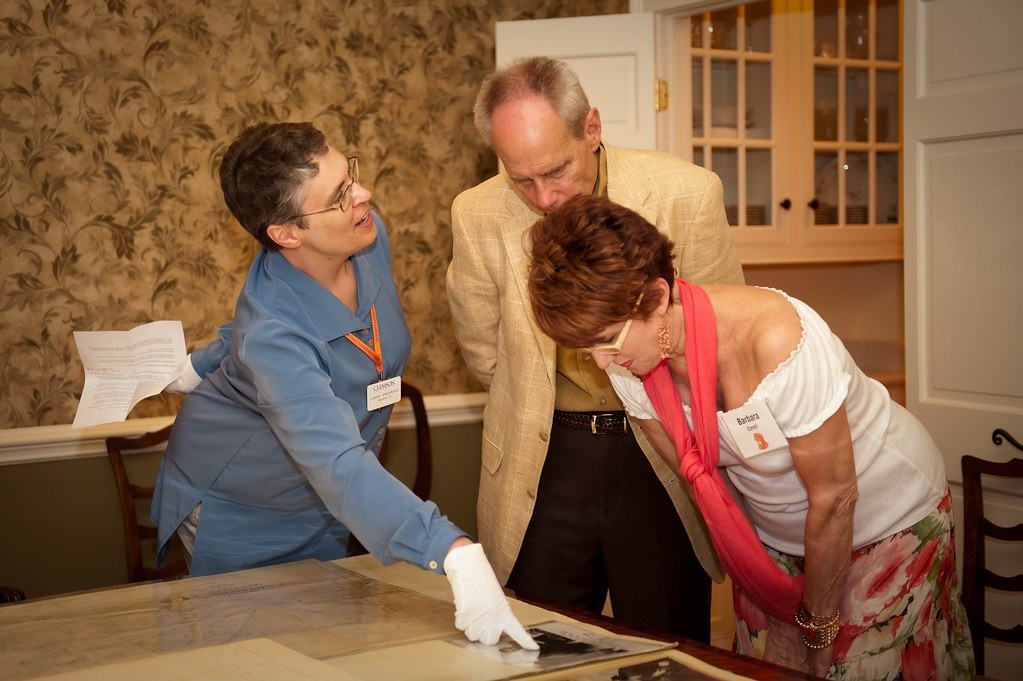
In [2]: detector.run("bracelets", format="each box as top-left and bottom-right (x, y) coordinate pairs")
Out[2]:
(794, 602), (840, 649)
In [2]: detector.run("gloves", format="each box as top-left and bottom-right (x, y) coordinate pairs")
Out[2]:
(464, 637), (541, 670)
(442, 543), (540, 651)
(159, 352), (203, 394)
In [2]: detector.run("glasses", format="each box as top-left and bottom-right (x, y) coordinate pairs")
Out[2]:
(284, 156), (361, 226)
(578, 286), (648, 354)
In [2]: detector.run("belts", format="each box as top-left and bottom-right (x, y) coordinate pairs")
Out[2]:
(552, 403), (629, 436)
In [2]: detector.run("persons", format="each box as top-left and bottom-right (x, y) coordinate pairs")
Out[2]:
(149, 122), (540, 650)
(521, 195), (980, 680)
(447, 57), (747, 650)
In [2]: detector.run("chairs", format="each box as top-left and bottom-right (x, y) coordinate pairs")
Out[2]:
(960, 427), (1023, 681)
(348, 382), (432, 557)
(107, 422), (179, 585)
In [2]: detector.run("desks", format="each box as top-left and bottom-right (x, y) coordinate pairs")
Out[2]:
(0, 551), (840, 681)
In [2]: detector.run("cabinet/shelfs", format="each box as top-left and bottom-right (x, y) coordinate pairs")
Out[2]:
(686, 0), (904, 269)
